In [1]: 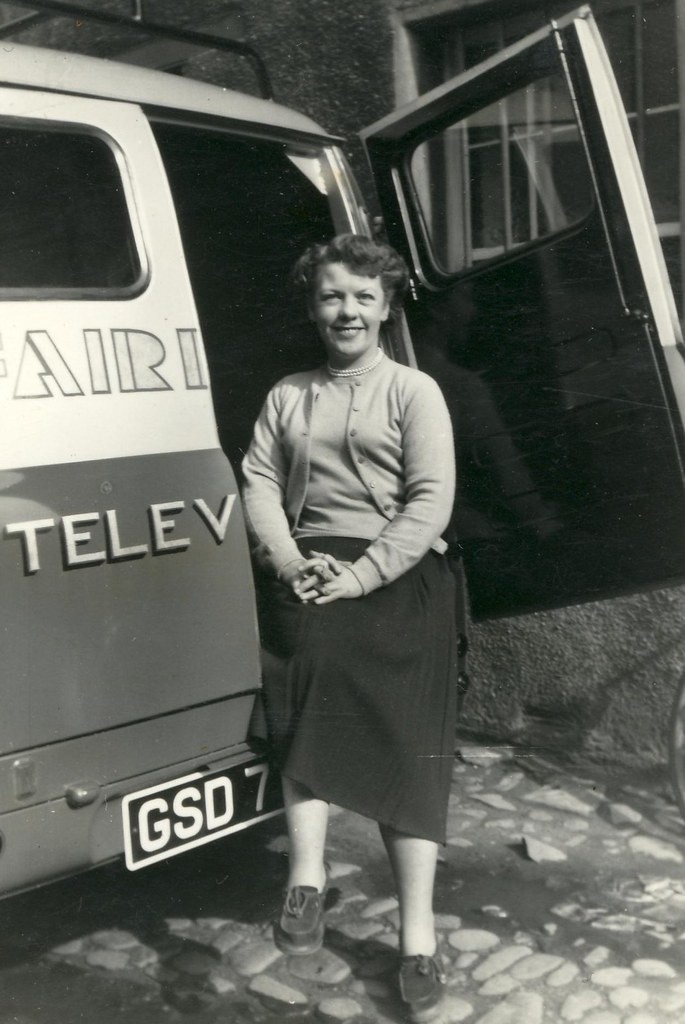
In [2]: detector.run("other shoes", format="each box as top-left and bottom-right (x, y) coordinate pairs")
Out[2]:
(394, 928), (448, 1021)
(275, 861), (332, 956)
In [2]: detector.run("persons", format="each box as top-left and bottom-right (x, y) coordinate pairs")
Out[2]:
(242, 234), (460, 1011)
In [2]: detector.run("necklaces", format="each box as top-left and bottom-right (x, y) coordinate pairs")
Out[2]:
(328, 346), (383, 376)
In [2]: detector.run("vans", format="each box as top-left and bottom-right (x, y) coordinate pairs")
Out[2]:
(0, 0), (684, 905)
